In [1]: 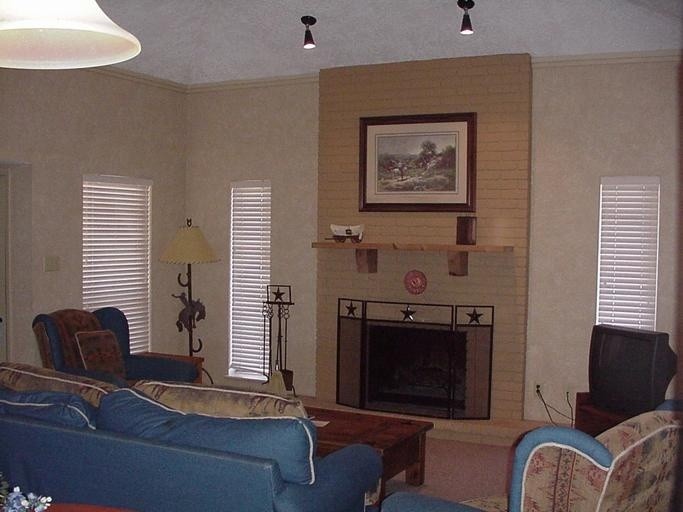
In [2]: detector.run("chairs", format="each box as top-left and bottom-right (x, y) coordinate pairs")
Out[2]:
(380, 399), (683, 512)
(32, 307), (203, 386)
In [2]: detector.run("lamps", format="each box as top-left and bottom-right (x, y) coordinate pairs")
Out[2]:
(301, 16), (316, 49)
(457, 0), (475, 35)
(0, 0), (142, 70)
(157, 217), (222, 358)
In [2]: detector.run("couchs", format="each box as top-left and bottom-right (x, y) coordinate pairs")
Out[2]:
(0, 361), (383, 512)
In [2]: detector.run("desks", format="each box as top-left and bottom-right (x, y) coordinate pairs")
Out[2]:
(574, 393), (633, 438)
(30, 502), (141, 512)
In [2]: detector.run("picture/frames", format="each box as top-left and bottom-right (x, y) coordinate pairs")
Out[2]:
(359, 112), (476, 211)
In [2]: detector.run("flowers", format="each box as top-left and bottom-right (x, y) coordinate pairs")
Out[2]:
(0, 485), (52, 512)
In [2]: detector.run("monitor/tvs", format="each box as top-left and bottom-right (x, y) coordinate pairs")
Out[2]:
(589, 324), (677, 417)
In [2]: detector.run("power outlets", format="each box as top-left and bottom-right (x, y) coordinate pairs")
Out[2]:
(534, 382), (545, 399)
(564, 387), (575, 400)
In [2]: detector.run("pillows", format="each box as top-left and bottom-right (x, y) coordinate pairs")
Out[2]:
(72, 331), (126, 380)
(96, 388), (320, 487)
(0, 388), (97, 431)
(0, 363), (122, 409)
(130, 378), (309, 419)
